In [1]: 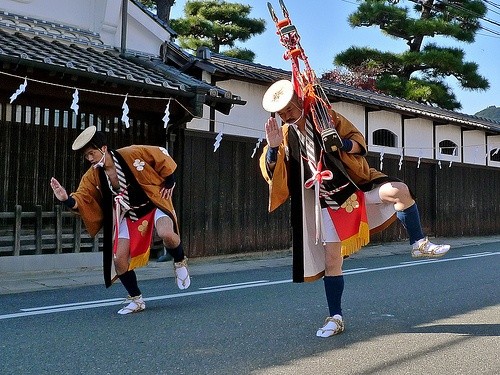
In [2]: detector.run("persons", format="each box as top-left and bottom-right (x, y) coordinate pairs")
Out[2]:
(50, 126), (190, 315)
(259, 80), (451, 337)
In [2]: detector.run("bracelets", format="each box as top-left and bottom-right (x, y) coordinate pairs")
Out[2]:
(267, 147), (279, 163)
(61, 195), (76, 209)
(340, 138), (353, 152)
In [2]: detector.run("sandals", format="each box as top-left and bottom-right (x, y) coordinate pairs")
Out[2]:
(411, 237), (445, 257)
(174, 255), (190, 290)
(118, 294), (146, 315)
(316, 317), (344, 338)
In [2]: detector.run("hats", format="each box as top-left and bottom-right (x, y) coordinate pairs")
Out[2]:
(262, 80), (303, 113)
(72, 126), (97, 151)
